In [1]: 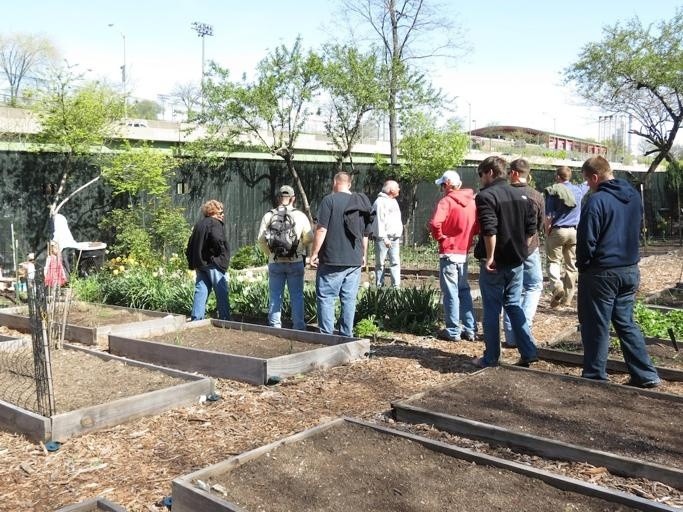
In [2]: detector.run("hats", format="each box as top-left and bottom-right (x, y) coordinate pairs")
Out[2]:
(279, 185), (294, 197)
(435, 170), (460, 186)
(203, 200), (222, 217)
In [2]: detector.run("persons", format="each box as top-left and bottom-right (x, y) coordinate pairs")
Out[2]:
(503, 159), (545, 348)
(186, 198), (231, 322)
(45, 239), (66, 296)
(310, 171), (369, 338)
(574, 154), (662, 389)
(543, 166), (593, 309)
(367, 180), (404, 290)
(474, 156), (539, 367)
(258, 185), (314, 330)
(428, 170), (480, 343)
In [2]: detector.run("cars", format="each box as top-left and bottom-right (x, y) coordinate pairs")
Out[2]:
(120, 118), (148, 129)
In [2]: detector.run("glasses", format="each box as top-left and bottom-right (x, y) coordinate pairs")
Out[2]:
(217, 211), (223, 215)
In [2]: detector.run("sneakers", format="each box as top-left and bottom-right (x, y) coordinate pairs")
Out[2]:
(515, 352), (538, 367)
(551, 288), (564, 307)
(623, 378), (661, 388)
(472, 358), (500, 368)
(439, 330), (478, 342)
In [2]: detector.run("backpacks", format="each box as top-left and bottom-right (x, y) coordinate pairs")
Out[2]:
(264, 208), (302, 261)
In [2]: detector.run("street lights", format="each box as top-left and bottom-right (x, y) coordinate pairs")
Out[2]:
(106, 20), (127, 117)
(190, 20), (215, 127)
